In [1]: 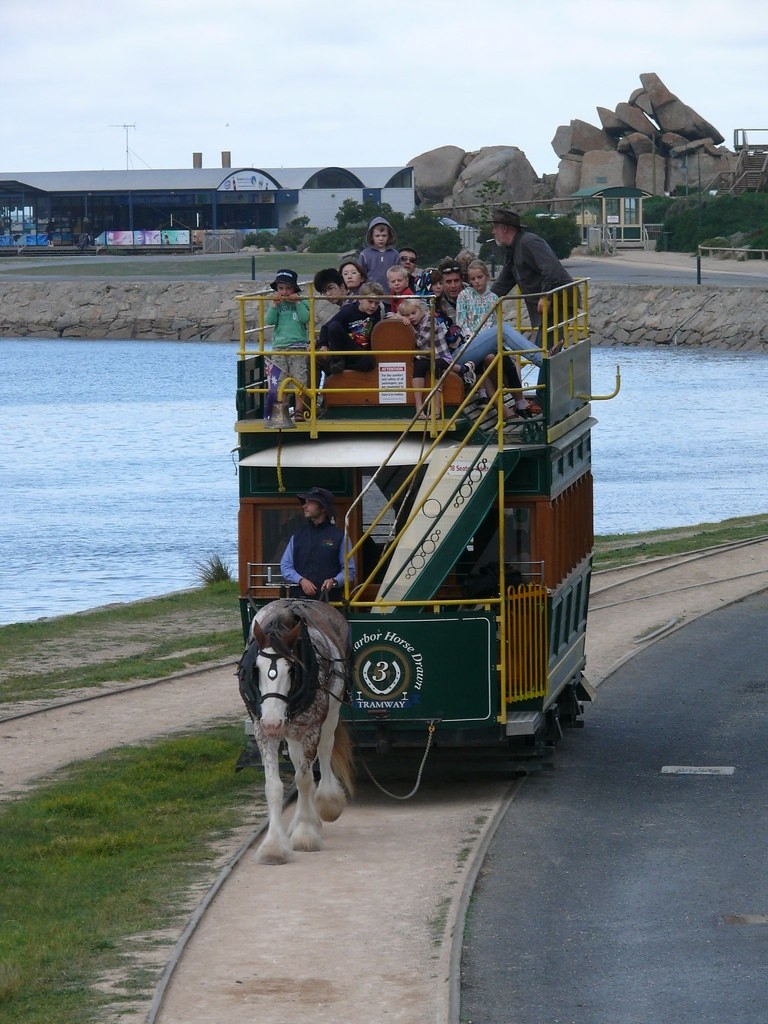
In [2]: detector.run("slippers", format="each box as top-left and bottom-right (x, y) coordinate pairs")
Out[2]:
(504, 414), (524, 423)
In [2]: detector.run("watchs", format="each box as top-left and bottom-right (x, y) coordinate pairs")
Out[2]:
(329, 578), (338, 588)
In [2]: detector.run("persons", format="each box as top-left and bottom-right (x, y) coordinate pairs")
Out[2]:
(264, 269), (311, 423)
(304, 247), (565, 421)
(489, 209), (582, 415)
(360, 217), (402, 312)
(280, 487), (356, 615)
(45, 218), (57, 247)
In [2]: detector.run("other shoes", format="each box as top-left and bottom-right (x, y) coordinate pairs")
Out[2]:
(515, 399), (543, 413)
(549, 339), (564, 357)
(428, 413), (441, 420)
(417, 413), (426, 420)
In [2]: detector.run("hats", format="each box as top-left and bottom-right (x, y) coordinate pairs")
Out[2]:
(270, 269), (301, 293)
(296, 487), (338, 516)
(485, 209), (528, 228)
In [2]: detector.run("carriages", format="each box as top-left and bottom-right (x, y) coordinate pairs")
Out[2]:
(234, 282), (622, 866)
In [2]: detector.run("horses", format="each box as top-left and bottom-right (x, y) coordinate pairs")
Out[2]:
(232, 593), (358, 865)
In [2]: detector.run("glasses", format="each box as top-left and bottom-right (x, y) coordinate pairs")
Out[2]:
(320, 285), (338, 295)
(342, 270), (361, 278)
(442, 267), (460, 274)
(400, 255), (416, 264)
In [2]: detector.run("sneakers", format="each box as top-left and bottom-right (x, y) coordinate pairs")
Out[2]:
(463, 361), (476, 389)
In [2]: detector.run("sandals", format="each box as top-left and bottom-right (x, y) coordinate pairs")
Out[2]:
(294, 410), (306, 422)
